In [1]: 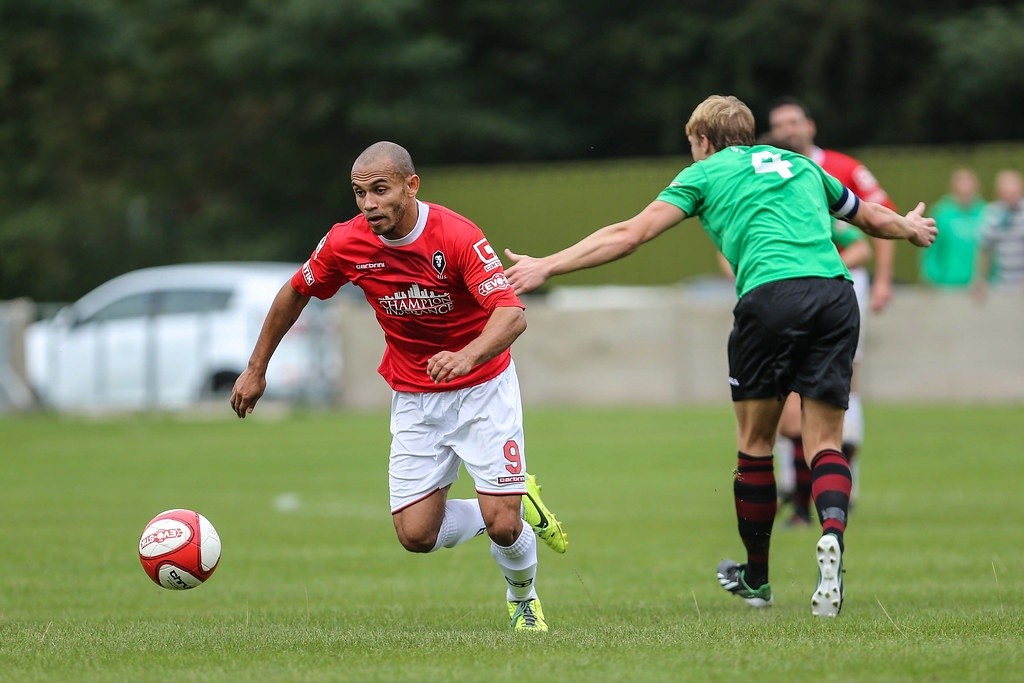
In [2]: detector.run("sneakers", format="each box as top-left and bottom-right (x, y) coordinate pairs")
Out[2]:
(520, 472), (569, 554)
(508, 600), (548, 637)
(717, 556), (771, 609)
(809, 531), (843, 619)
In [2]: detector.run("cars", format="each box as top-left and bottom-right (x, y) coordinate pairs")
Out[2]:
(23, 261), (341, 419)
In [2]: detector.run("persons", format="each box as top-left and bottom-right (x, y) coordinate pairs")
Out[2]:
(502, 94), (938, 619)
(754, 97), (898, 530)
(980, 169), (1024, 285)
(917, 167), (988, 288)
(230, 140), (567, 631)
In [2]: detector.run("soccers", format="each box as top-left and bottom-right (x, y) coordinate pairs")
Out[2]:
(136, 507), (224, 591)
